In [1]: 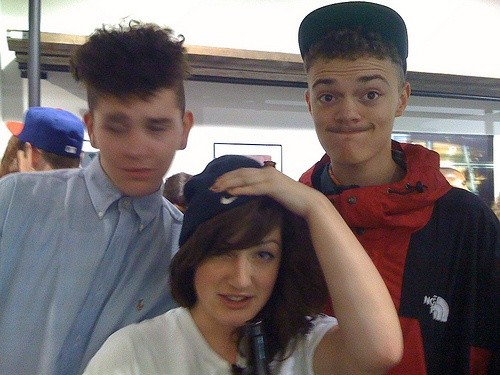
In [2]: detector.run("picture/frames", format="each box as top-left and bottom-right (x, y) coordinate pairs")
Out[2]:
(214, 143), (282, 172)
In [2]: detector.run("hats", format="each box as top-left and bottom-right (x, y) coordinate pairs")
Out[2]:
(7, 107), (85, 158)
(179, 154), (264, 248)
(298, 1), (408, 61)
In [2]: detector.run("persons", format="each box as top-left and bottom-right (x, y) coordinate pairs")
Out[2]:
(82, 155), (404, 375)
(0, 106), (85, 174)
(0, 21), (194, 375)
(297, 1), (500, 375)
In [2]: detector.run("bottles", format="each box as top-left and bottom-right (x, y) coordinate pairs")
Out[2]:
(243, 318), (271, 375)
